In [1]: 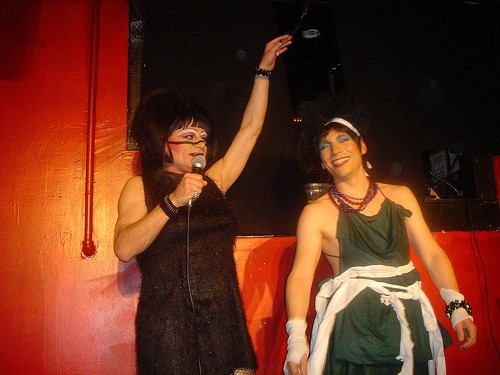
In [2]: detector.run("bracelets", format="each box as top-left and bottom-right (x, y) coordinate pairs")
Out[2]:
(255, 65), (274, 78)
(445, 299), (473, 318)
(159, 194), (178, 218)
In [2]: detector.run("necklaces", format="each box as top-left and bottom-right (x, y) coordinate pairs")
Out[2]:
(328, 179), (379, 213)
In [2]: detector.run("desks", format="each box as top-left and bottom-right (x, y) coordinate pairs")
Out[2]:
(423, 198), (479, 229)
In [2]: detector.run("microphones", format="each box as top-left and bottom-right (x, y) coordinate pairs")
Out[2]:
(430, 171), (464, 200)
(187, 155), (207, 207)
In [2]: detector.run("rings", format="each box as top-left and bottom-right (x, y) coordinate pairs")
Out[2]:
(276, 51), (280, 57)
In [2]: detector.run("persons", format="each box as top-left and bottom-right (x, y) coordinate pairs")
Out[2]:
(283, 113), (478, 375)
(113, 34), (296, 375)
